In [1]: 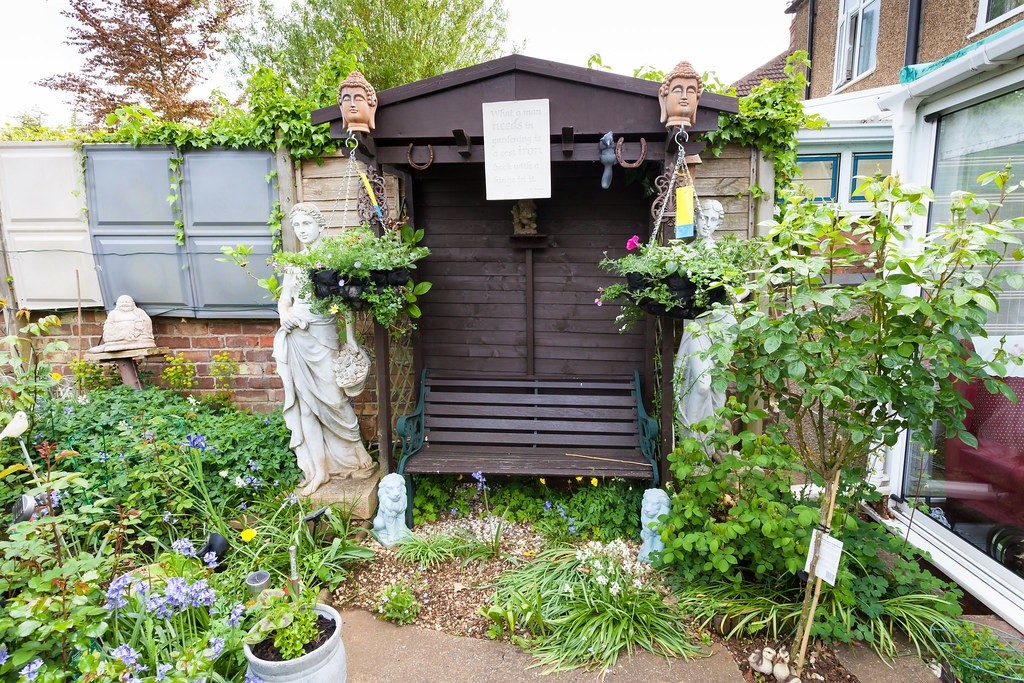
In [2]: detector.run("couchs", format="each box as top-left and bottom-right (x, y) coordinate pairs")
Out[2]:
(940, 332), (1024, 535)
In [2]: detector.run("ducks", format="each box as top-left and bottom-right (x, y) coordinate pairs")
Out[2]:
(744, 646), (790, 683)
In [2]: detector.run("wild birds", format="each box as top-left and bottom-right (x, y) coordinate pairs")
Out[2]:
(0, 411), (28, 440)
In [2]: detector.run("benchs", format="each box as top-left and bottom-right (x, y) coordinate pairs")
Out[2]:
(394, 367), (659, 531)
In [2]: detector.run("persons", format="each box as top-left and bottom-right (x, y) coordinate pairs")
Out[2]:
(103, 295), (155, 342)
(337, 72), (378, 133)
(673, 199), (750, 459)
(270, 201), (373, 496)
(658, 60), (702, 127)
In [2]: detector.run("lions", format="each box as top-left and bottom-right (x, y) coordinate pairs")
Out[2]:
(373, 472), (408, 542)
(639, 488), (671, 560)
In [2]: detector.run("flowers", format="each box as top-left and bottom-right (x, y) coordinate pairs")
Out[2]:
(593, 234), (769, 336)
(267, 220), (431, 334)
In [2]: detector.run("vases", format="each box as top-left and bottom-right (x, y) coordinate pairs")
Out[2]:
(307, 267), (419, 312)
(625, 269), (724, 321)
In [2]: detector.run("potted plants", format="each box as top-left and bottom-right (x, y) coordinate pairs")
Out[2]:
(243, 584), (348, 683)
